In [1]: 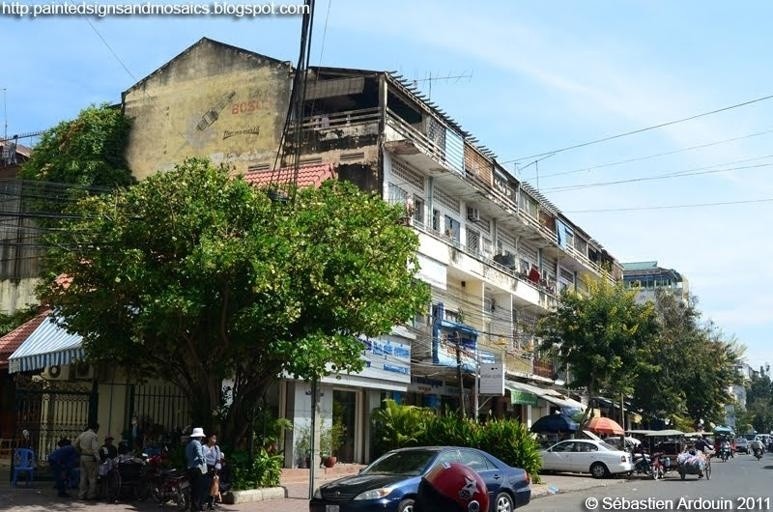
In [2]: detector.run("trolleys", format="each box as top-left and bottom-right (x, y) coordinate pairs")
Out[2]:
(676, 446), (716, 483)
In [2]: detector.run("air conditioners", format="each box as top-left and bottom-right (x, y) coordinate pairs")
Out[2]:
(75, 361), (94, 379)
(467, 205), (480, 222)
(44, 365), (73, 380)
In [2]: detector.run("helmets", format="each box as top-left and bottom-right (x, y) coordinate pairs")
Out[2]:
(421, 461), (491, 512)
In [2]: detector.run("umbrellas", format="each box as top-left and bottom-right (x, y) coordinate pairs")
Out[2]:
(530, 413), (578, 444)
(584, 416), (624, 439)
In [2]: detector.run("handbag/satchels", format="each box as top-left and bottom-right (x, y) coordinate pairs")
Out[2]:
(208, 472), (222, 496)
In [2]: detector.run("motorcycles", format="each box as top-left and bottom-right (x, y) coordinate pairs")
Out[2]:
(715, 432), (731, 462)
(754, 444), (763, 460)
(63, 446), (231, 510)
(616, 440), (667, 480)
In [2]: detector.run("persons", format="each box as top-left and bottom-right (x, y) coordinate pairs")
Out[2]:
(694, 434), (773, 477)
(131, 415), (145, 449)
(200, 431), (224, 512)
(45, 422), (118, 501)
(185, 427), (207, 512)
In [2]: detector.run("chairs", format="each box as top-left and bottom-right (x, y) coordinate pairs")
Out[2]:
(11, 448), (37, 488)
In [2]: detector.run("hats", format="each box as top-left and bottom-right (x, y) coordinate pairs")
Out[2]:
(190, 427), (207, 438)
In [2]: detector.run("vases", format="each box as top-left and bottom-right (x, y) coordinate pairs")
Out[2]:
(324, 457), (336, 467)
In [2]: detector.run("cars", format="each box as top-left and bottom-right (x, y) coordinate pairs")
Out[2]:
(308, 445), (531, 511)
(688, 439), (715, 458)
(734, 437), (751, 454)
(754, 433), (771, 447)
(531, 439), (630, 480)
